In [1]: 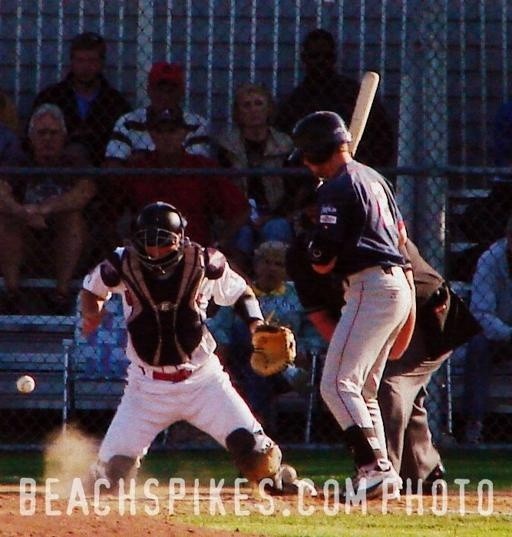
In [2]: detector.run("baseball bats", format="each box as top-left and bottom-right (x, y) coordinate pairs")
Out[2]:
(348, 71), (380, 159)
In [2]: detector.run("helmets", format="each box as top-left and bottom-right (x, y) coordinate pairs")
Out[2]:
(275, 174), (319, 239)
(286, 111), (353, 165)
(129, 202), (185, 270)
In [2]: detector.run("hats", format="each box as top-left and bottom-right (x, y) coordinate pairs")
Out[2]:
(148, 61), (184, 88)
(146, 103), (185, 128)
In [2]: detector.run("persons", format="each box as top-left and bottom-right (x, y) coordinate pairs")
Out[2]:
(453, 215), (511, 452)
(281, 147), (452, 498)
(284, 109), (412, 508)
(1, 24), (389, 436)
(77, 201), (316, 499)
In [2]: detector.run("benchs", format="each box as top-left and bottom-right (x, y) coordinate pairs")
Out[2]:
(0, 242), (512, 416)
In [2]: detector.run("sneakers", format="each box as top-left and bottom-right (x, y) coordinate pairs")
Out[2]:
(339, 461), (443, 504)
(260, 479), (318, 498)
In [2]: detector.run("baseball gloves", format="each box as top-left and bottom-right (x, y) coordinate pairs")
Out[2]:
(249, 324), (297, 377)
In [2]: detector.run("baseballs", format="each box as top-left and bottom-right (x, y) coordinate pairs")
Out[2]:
(17, 374), (37, 393)
(282, 465), (299, 484)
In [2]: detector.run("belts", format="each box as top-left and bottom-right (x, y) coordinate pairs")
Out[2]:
(138, 364), (192, 383)
(344, 262), (391, 287)
(419, 279), (450, 314)
